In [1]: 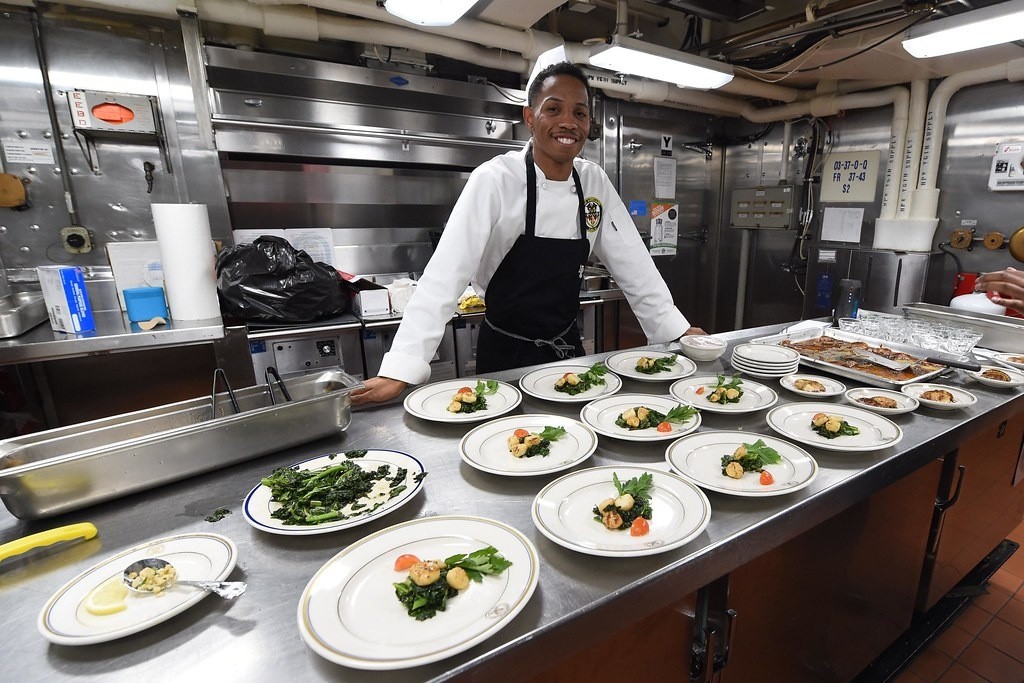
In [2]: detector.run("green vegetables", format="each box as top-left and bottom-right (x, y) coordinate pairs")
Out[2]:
(204, 449), (430, 526)
(456, 353), (861, 530)
(393, 545), (514, 621)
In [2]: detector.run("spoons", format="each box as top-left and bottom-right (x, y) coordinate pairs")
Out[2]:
(121, 558), (248, 601)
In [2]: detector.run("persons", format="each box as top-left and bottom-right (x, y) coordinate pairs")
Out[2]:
(350, 61), (710, 405)
(975, 267), (1024, 318)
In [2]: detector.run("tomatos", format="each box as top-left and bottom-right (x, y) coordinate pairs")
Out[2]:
(657, 422), (672, 432)
(514, 429), (530, 437)
(696, 387), (704, 394)
(395, 554), (420, 571)
(630, 516), (649, 536)
(760, 471), (774, 484)
(458, 387), (471, 393)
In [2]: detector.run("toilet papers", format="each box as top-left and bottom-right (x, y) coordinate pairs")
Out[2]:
(152, 202), (220, 322)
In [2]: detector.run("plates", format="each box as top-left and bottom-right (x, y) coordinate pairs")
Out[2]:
(518, 364), (624, 403)
(296, 514), (539, 670)
(992, 352), (1024, 369)
(844, 387), (919, 416)
(668, 375), (779, 415)
(605, 350), (698, 382)
(579, 393), (702, 442)
(664, 429), (819, 498)
(530, 464), (712, 558)
(730, 343), (800, 380)
(765, 401), (904, 452)
(780, 374), (847, 398)
(242, 448), (426, 536)
(963, 365), (1024, 389)
(901, 382), (978, 411)
(37, 532), (238, 647)
(402, 377), (523, 423)
(458, 414), (599, 477)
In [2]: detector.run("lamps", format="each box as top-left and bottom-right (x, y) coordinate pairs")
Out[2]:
(376, 0), (479, 27)
(589, 34), (734, 89)
(901, 0), (1024, 58)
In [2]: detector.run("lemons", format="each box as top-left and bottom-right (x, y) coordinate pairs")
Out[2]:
(83, 577), (128, 614)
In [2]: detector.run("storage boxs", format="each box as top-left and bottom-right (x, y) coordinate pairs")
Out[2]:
(349, 276), (390, 316)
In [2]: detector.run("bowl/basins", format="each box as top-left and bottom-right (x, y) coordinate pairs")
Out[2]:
(679, 334), (728, 362)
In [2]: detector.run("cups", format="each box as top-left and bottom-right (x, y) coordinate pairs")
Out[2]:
(838, 314), (984, 362)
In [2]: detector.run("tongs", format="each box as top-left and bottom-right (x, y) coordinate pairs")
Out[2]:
(265, 366), (293, 406)
(210, 367), (242, 422)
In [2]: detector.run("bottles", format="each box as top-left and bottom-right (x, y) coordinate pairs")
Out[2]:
(654, 219), (663, 242)
(833, 279), (862, 327)
